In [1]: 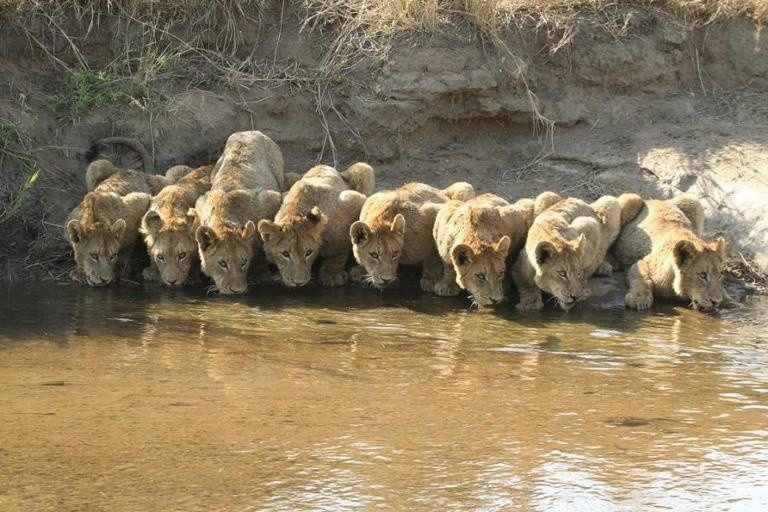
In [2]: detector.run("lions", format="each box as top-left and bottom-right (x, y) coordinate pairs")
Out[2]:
(59, 127), (743, 313)
